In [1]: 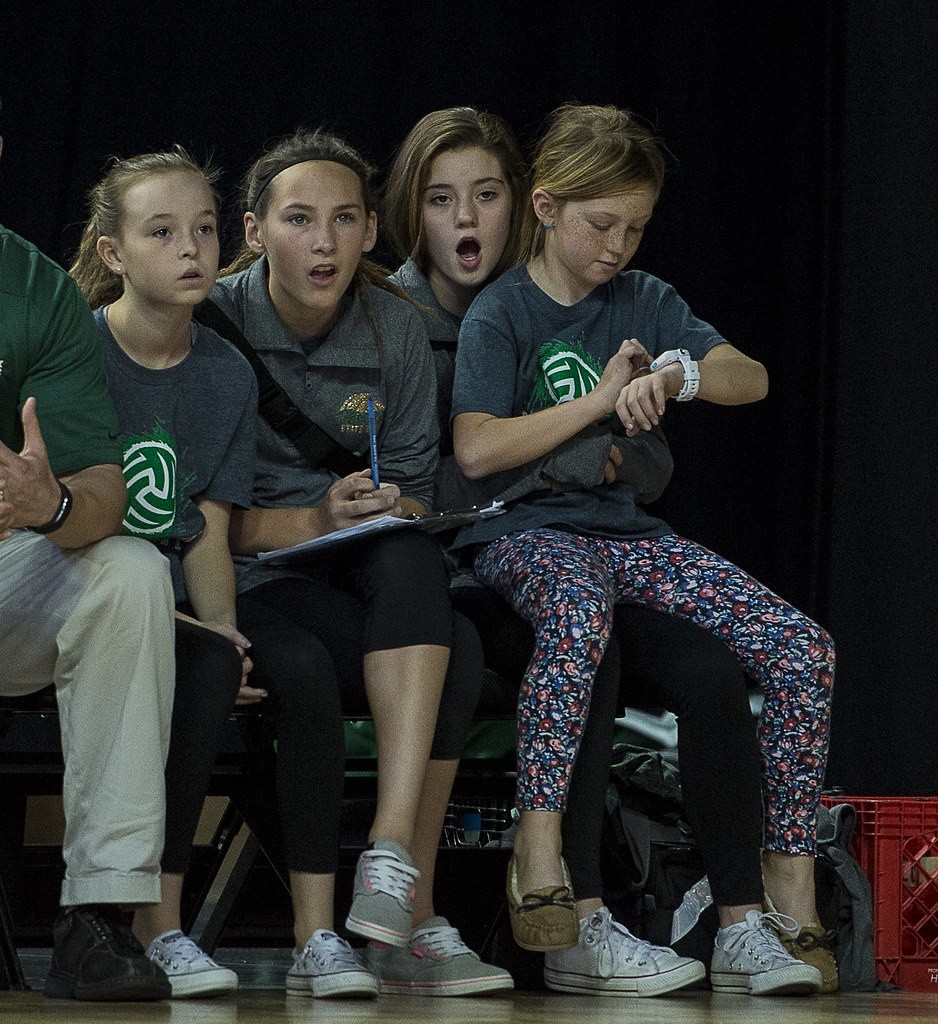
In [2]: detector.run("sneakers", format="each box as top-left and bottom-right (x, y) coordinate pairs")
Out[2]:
(285, 929), (380, 999)
(144, 928), (239, 998)
(710, 909), (823, 996)
(505, 854), (580, 952)
(367, 915), (514, 996)
(761, 876), (840, 992)
(346, 836), (421, 950)
(543, 906), (706, 998)
(42, 905), (172, 1000)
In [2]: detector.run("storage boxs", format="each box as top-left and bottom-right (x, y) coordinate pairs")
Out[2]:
(820, 796), (938, 994)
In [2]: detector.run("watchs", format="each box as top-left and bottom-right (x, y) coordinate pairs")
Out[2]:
(649, 347), (701, 404)
(11, 478), (74, 534)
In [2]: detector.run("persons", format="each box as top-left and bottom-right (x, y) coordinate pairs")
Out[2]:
(0, 93), (835, 1002)
